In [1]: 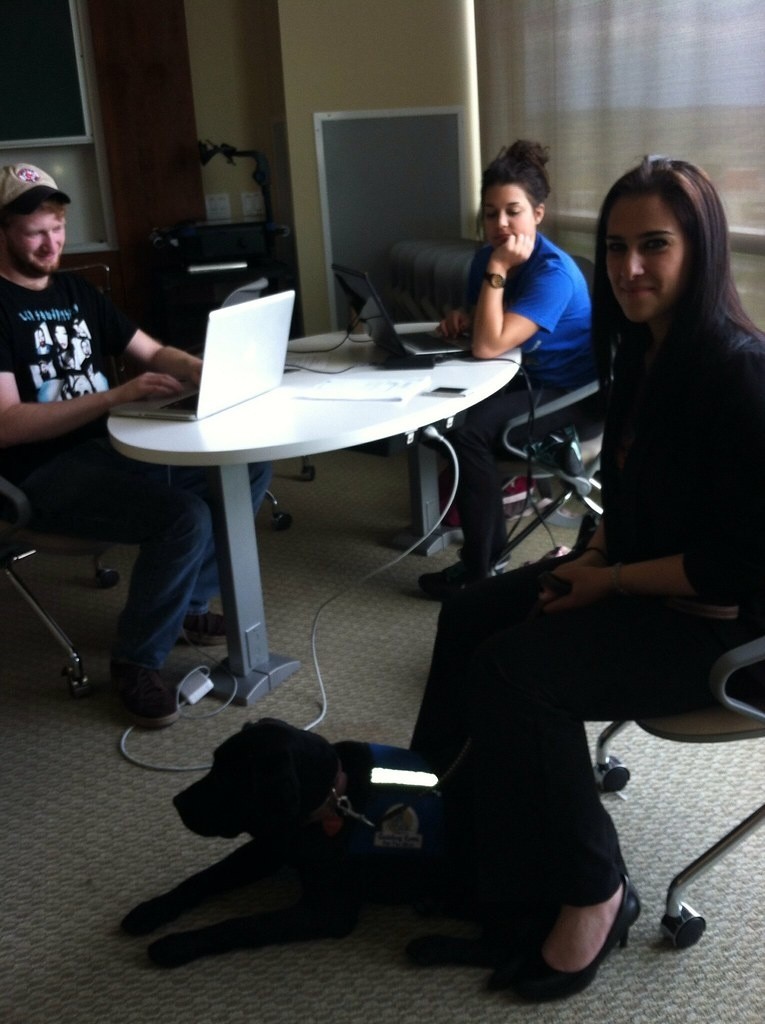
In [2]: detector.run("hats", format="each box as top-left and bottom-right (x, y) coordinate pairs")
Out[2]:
(0, 163), (71, 214)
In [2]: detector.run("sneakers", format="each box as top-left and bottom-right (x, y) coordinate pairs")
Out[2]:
(180, 612), (229, 645)
(110, 660), (176, 727)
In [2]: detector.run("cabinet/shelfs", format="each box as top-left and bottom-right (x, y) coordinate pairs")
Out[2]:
(178, 219), (302, 356)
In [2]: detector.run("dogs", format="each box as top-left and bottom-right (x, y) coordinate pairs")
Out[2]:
(119, 718), (552, 969)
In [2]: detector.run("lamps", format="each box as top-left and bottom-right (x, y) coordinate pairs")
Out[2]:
(199, 138), (279, 288)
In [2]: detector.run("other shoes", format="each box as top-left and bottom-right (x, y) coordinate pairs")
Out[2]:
(418, 563), (483, 600)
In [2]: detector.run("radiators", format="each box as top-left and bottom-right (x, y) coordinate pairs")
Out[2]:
(390, 236), (597, 320)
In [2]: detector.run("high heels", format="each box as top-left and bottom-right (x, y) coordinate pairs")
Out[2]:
(506, 876), (641, 1002)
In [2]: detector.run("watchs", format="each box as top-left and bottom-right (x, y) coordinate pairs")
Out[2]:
(482, 272), (506, 288)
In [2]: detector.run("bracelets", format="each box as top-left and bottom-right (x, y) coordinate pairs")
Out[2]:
(586, 547), (606, 555)
(613, 563), (623, 596)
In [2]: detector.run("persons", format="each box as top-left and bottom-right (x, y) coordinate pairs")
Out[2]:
(0, 162), (270, 727)
(416, 140), (593, 592)
(407, 158), (765, 1002)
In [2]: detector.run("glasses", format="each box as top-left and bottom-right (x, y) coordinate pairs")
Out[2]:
(538, 571), (572, 597)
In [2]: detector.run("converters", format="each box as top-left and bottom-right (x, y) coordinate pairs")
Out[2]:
(176, 670), (214, 705)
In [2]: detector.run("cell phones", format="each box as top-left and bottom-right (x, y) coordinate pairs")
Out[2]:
(423, 387), (469, 397)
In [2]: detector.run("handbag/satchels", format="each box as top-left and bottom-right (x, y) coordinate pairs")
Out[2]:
(439, 465), (536, 527)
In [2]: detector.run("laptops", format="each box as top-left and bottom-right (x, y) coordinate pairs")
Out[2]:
(108, 286), (297, 421)
(330, 263), (479, 363)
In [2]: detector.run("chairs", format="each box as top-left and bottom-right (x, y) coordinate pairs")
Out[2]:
(0, 253), (764, 951)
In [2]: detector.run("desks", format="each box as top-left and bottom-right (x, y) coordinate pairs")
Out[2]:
(108, 321), (526, 704)
